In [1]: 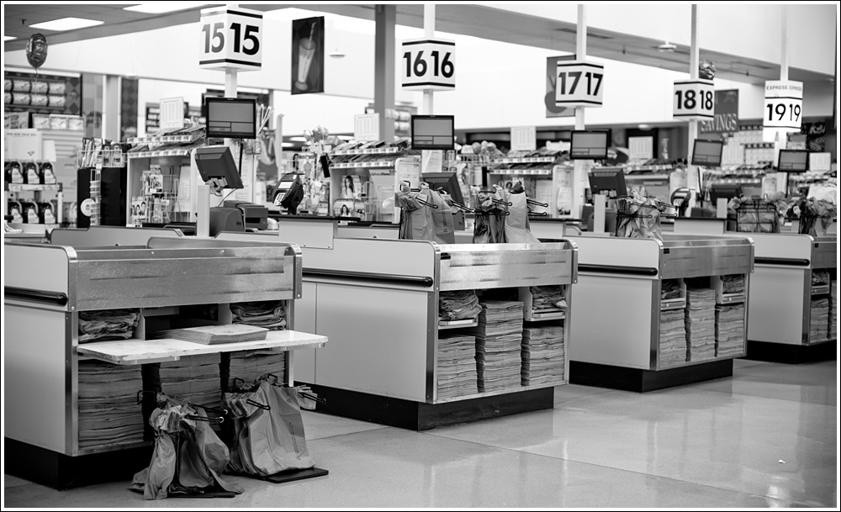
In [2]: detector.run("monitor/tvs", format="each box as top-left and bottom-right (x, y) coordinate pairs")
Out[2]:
(410, 115), (453, 149)
(570, 132), (608, 158)
(195, 147), (244, 189)
(692, 138), (723, 166)
(204, 98), (255, 138)
(422, 172), (464, 206)
(777, 149), (809, 172)
(709, 184), (742, 207)
(589, 167), (627, 199)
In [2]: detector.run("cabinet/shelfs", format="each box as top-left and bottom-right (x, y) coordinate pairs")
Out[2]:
(442, 152), (576, 218)
(661, 218), (837, 365)
(77, 137), (267, 227)
(2, 68), (87, 226)
(216, 219), (578, 432)
(453, 217), (755, 395)
(613, 160), (837, 211)
(4, 227), (327, 491)
(294, 141), (420, 226)
